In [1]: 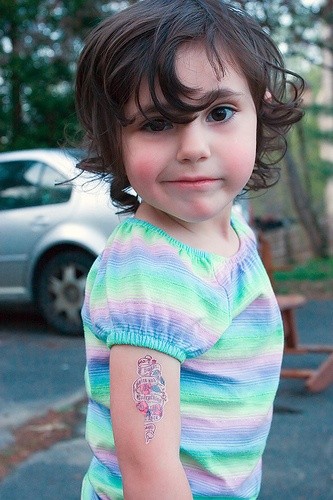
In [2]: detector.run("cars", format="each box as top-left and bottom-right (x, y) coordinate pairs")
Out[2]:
(0, 148), (143, 336)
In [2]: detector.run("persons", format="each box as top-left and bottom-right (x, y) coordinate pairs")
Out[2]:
(64, 2), (307, 500)
